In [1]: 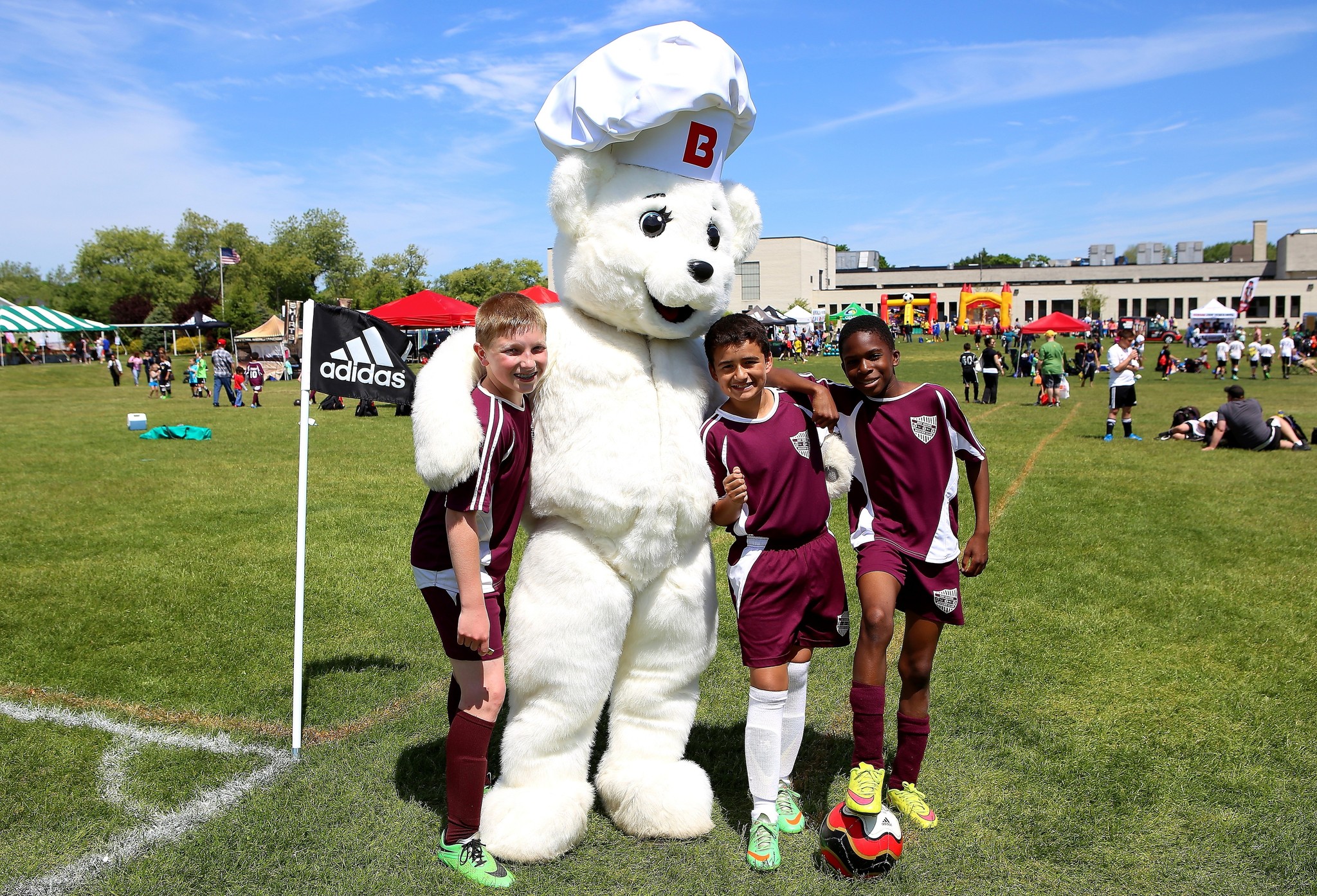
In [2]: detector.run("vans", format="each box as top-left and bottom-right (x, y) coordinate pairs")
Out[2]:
(1116, 316), (1182, 344)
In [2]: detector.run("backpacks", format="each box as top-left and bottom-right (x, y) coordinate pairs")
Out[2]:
(1173, 405), (1200, 425)
(355, 399), (378, 415)
(320, 395), (344, 410)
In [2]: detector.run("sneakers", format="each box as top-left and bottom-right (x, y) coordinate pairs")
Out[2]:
(436, 828), (515, 889)
(844, 760), (886, 815)
(1104, 434), (1112, 441)
(1124, 433), (1142, 441)
(746, 818), (781, 870)
(775, 783), (806, 833)
(887, 781), (938, 829)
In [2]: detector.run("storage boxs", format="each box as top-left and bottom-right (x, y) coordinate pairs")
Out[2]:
(126, 412), (148, 431)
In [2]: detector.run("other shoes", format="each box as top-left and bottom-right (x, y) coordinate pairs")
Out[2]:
(1047, 402), (1053, 407)
(1214, 372), (1290, 380)
(1055, 402), (1060, 407)
(1292, 442), (1310, 451)
(213, 402), (261, 408)
(982, 401), (988, 404)
(973, 399), (980, 403)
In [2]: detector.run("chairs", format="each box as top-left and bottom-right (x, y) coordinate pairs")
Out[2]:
(1288, 361), (1309, 375)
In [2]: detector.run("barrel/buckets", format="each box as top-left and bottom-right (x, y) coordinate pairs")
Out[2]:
(339, 298), (353, 308)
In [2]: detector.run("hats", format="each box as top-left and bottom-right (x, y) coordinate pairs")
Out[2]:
(1044, 330), (1055, 337)
(218, 339), (226, 345)
(1224, 385), (1244, 398)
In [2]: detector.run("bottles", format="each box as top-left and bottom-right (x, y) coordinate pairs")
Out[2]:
(1277, 410), (1283, 418)
(1014, 373), (1016, 377)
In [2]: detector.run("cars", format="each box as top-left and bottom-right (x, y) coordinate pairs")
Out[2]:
(404, 329), (451, 365)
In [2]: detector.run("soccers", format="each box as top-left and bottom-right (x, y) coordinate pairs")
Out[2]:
(827, 352), (831, 356)
(293, 399), (301, 406)
(835, 348), (839, 353)
(818, 801), (903, 884)
(825, 349), (829, 353)
(831, 352), (835, 356)
(830, 348), (835, 352)
(834, 353), (840, 356)
(823, 352), (827, 356)
(824, 345), (829, 348)
(1135, 374), (1143, 380)
(828, 347), (831, 350)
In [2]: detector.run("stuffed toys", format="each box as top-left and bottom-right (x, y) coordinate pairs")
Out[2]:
(411, 20), (764, 862)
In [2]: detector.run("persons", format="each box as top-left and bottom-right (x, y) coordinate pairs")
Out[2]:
(960, 311), (1317, 451)
(764, 322), (840, 364)
(699, 314), (851, 872)
(411, 293), (549, 889)
(108, 337), (266, 409)
(68, 334), (112, 365)
(885, 315), (958, 343)
(765, 315), (991, 828)
(3, 336), (37, 366)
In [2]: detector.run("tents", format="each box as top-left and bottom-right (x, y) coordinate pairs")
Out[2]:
(519, 285), (557, 304)
(1190, 299), (1238, 331)
(1011, 311), (1091, 380)
(828, 302), (879, 335)
(784, 306), (813, 323)
(163, 311), (234, 355)
(0, 304), (120, 368)
(762, 306), (797, 340)
(745, 306), (785, 325)
(365, 288), (478, 328)
(233, 314), (302, 380)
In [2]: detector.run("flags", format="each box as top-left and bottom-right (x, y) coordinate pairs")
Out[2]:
(301, 300), (416, 410)
(220, 246), (240, 266)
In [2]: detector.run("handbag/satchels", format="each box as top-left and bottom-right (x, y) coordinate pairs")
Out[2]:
(1059, 376), (1070, 399)
(975, 353), (983, 372)
